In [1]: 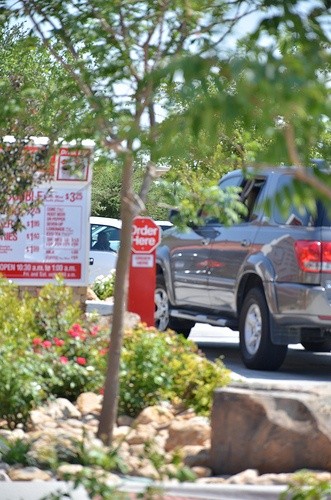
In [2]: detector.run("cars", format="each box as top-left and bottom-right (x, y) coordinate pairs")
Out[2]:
(88, 216), (123, 288)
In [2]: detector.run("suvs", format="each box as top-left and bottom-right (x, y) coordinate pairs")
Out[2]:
(152, 159), (331, 371)
